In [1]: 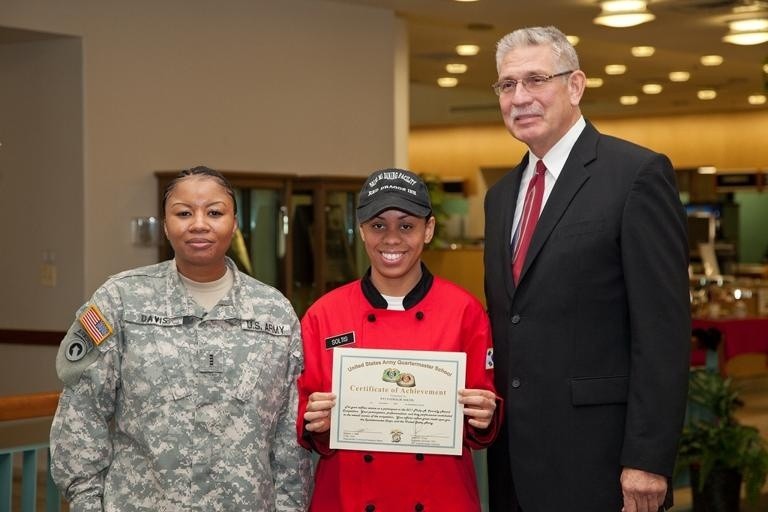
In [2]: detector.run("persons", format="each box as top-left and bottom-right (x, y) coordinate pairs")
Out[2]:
(294, 166), (504, 511)
(46, 165), (316, 512)
(482, 24), (694, 510)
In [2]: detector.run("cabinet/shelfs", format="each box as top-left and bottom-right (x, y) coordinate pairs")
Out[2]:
(154, 172), (364, 321)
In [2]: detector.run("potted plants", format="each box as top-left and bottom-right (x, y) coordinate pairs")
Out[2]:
(662, 366), (768, 512)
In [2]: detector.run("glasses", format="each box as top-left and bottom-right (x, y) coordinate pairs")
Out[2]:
(492, 66), (574, 98)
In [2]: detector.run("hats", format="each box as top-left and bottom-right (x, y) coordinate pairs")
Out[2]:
(357, 168), (432, 225)
